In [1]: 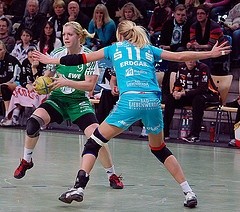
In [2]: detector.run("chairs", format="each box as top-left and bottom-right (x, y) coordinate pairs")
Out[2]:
(155, 71), (238, 147)
(141, 0), (240, 69)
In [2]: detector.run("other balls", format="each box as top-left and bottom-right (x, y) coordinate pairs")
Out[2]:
(32, 74), (53, 97)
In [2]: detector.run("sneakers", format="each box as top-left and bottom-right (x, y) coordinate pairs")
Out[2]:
(14, 158), (33, 179)
(109, 172), (124, 189)
(58, 187), (84, 204)
(182, 191), (198, 207)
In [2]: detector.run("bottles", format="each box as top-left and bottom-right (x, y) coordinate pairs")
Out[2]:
(180, 118), (191, 138)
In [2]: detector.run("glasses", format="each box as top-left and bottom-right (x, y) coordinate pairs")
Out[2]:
(197, 12), (206, 15)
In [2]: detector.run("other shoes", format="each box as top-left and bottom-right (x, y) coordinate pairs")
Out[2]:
(0, 115), (19, 127)
(183, 135), (201, 143)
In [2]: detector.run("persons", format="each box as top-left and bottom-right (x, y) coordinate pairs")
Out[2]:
(0, 0), (240, 143)
(14, 21), (124, 189)
(31, 20), (232, 208)
